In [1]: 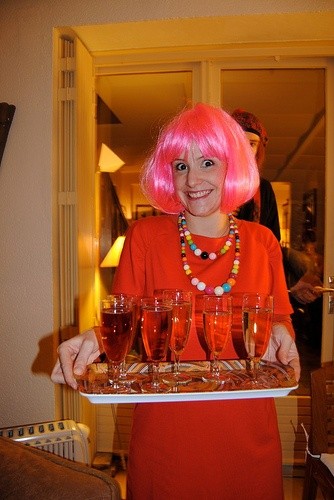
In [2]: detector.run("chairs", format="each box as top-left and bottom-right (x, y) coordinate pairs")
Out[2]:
(301, 360), (334, 500)
(0, 435), (121, 500)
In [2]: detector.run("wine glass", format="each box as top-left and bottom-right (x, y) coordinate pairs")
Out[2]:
(200, 295), (234, 385)
(241, 293), (276, 385)
(98, 293), (139, 395)
(139, 298), (172, 393)
(162, 289), (194, 385)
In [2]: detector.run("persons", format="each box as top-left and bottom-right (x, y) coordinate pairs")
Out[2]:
(230, 109), (318, 332)
(51, 103), (300, 500)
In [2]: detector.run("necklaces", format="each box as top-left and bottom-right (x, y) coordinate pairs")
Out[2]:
(178, 213), (240, 298)
